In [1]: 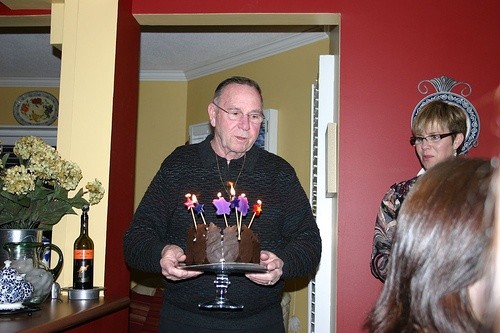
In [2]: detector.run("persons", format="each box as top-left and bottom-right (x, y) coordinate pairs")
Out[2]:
(123, 76), (322, 333)
(369, 102), (468, 283)
(357, 157), (500, 333)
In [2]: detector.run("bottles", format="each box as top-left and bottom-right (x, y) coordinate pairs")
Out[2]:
(73, 212), (95, 290)
(0, 259), (34, 312)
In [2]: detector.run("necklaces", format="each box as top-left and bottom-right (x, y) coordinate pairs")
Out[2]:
(215, 152), (246, 198)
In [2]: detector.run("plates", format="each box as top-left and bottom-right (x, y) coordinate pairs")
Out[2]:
(411, 91), (480, 155)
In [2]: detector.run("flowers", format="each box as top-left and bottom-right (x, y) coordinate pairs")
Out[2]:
(0, 135), (105, 227)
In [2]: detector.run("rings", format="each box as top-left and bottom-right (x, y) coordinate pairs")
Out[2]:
(265, 280), (272, 285)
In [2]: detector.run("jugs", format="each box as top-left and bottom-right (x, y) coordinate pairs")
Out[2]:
(1, 241), (64, 312)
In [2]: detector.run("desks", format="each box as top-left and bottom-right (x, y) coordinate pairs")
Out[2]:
(0, 294), (130, 333)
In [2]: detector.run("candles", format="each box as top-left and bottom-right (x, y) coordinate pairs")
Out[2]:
(228, 182), (241, 232)
(248, 198), (264, 227)
(212, 192), (232, 229)
(182, 190), (200, 229)
(236, 191), (251, 229)
(192, 194), (206, 223)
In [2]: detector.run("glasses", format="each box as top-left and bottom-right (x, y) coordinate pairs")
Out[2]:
(213, 102), (265, 124)
(410, 131), (459, 147)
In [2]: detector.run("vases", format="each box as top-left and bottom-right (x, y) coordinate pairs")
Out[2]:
(0, 228), (43, 267)
